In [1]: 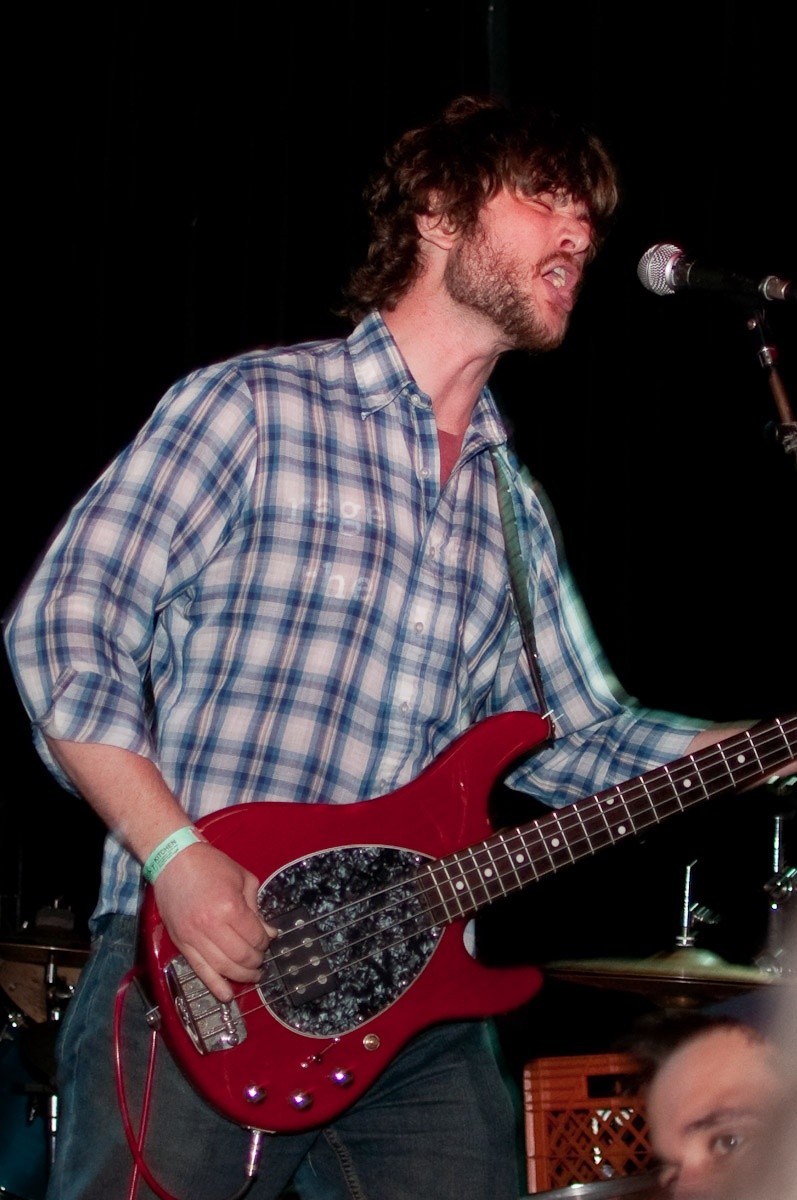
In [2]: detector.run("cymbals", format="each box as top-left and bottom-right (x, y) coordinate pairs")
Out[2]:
(544, 943), (794, 1012)
(0, 932), (97, 966)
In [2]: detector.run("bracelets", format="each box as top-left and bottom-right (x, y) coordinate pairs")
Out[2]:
(142, 824), (209, 889)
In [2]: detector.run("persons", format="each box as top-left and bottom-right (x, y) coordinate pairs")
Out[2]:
(3, 90), (794, 1200)
(646, 991), (797, 1198)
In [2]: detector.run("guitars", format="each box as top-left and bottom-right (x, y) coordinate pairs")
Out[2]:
(137, 709), (796, 1133)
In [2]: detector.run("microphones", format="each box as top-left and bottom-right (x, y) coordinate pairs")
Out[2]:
(635, 244), (797, 302)
